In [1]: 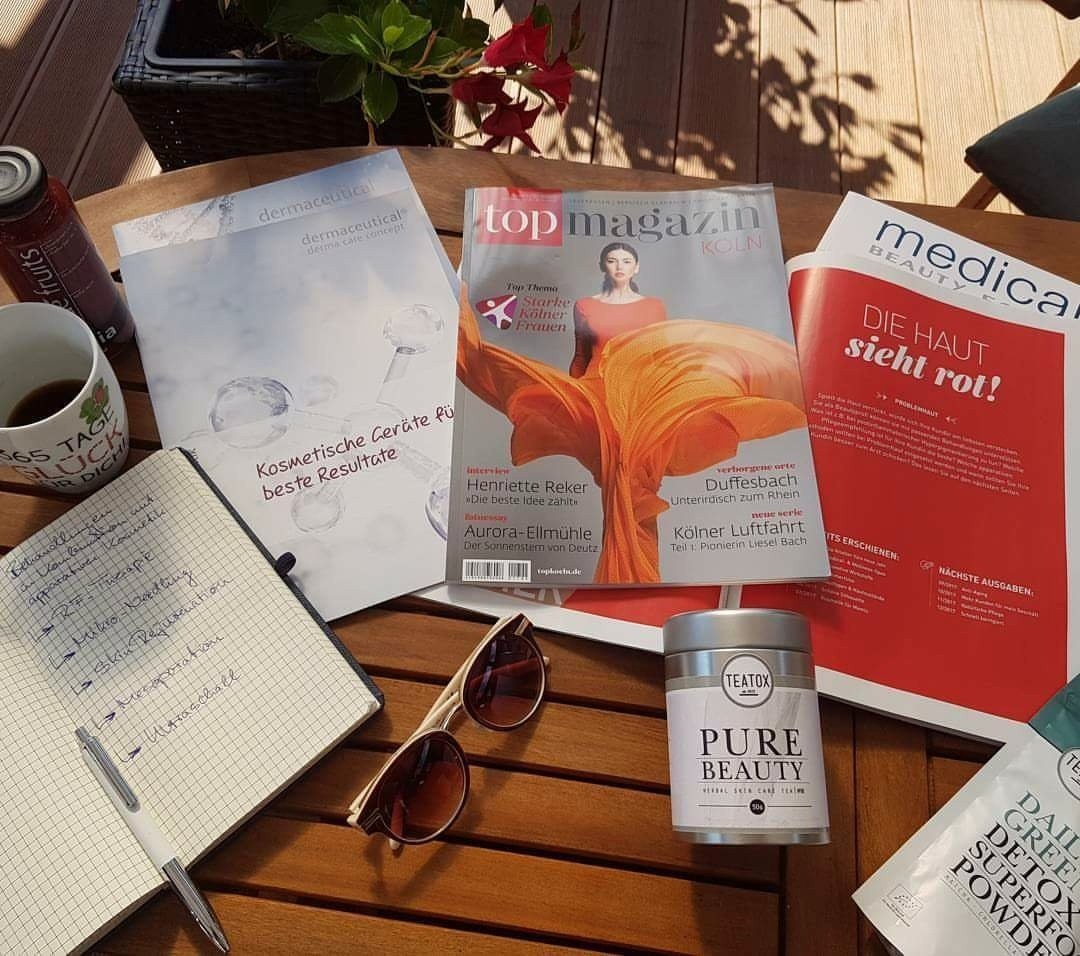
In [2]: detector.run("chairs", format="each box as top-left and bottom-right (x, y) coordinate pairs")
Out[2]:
(957, 57), (1080, 223)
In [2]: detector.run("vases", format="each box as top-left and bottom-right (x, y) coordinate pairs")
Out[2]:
(110, 0), (456, 172)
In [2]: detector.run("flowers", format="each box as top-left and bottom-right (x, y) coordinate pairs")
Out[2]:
(225, 0), (589, 152)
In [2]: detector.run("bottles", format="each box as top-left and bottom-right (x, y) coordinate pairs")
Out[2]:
(0, 146), (134, 360)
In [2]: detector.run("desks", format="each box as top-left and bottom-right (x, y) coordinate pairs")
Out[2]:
(0, 148), (1080, 956)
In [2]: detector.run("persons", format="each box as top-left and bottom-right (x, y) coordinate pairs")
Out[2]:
(455, 243), (810, 583)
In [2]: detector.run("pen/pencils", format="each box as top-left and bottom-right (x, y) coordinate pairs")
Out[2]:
(75, 727), (230, 953)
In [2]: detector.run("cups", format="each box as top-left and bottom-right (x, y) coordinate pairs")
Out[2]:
(0, 302), (130, 494)
